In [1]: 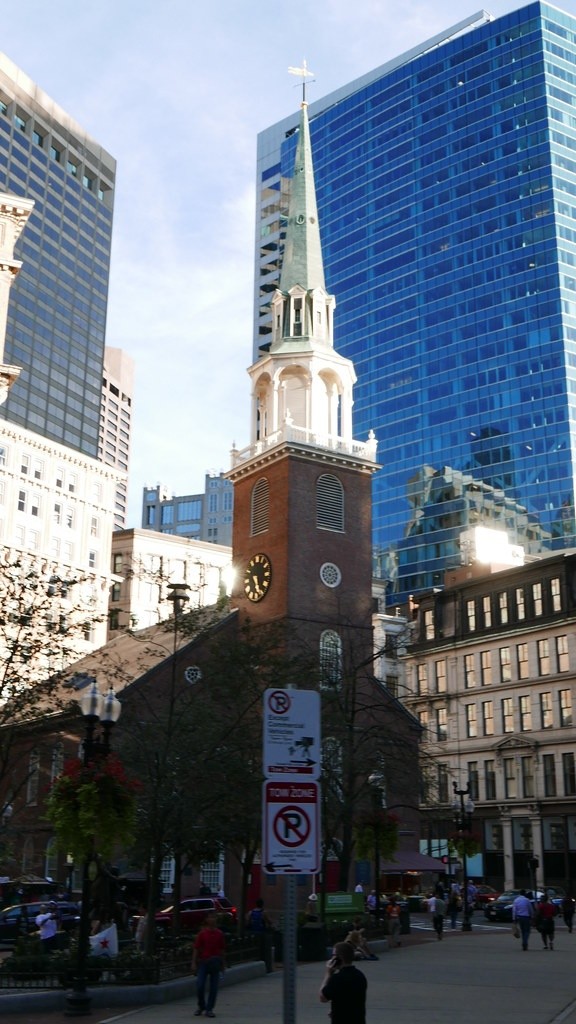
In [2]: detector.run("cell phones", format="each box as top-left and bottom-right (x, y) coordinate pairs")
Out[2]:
(331, 958), (342, 967)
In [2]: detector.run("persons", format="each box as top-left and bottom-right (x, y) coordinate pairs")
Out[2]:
(319, 942), (367, 1024)
(35, 904), (60, 955)
(191, 914), (231, 1018)
(449, 878), (479, 904)
(138, 901), (148, 916)
(245, 897), (272, 934)
(432, 892), (445, 940)
(434, 880), (445, 898)
(512, 888), (535, 951)
(561, 897), (576, 933)
(534, 894), (558, 950)
(448, 892), (459, 929)
(367, 889), (376, 915)
(386, 895), (402, 947)
(47, 900), (64, 931)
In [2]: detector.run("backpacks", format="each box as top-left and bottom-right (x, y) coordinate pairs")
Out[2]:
(250, 909), (263, 931)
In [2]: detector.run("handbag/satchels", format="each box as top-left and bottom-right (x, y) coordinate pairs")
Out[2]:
(512, 924), (520, 938)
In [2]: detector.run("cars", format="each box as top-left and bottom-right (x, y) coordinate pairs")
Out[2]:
(369, 881), (576, 924)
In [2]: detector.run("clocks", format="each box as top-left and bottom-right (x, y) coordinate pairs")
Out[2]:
(319, 562), (341, 587)
(243, 554), (272, 603)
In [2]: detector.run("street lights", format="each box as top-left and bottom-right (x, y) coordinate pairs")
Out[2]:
(63, 851), (77, 901)
(55, 678), (122, 1018)
(366, 768), (388, 930)
(141, 581), (193, 985)
(340, 643), (427, 891)
(449, 780), (476, 933)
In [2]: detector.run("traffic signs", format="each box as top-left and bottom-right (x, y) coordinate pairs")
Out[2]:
(260, 686), (323, 780)
(260, 777), (323, 876)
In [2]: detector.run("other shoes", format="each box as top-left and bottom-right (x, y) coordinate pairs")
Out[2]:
(550, 943), (553, 949)
(544, 947), (548, 949)
(206, 1011), (215, 1017)
(194, 1009), (203, 1015)
(364, 954), (378, 960)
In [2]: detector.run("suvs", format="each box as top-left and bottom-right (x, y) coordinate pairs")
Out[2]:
(0, 875), (72, 910)
(128, 895), (238, 945)
(0, 899), (83, 953)
